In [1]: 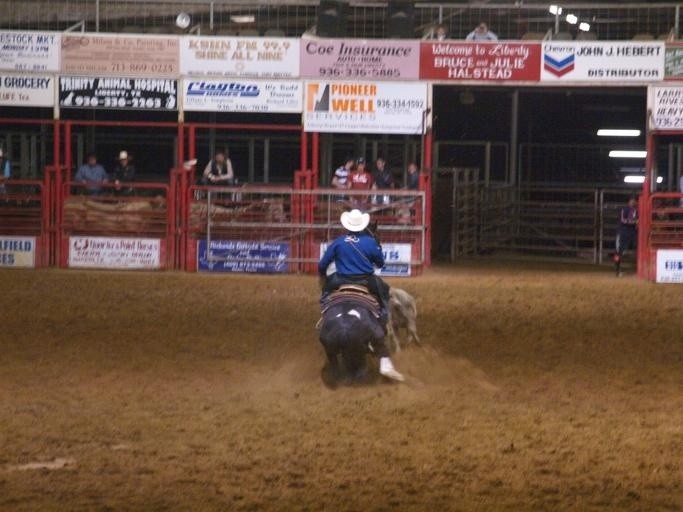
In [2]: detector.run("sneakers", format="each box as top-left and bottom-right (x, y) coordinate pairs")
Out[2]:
(378, 357), (406, 382)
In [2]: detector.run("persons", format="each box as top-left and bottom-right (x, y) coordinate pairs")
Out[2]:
(613, 196), (639, 276)
(203, 151), (234, 187)
(0, 146), (11, 193)
(315, 210), (389, 308)
(75, 150), (135, 194)
(331, 155), (420, 214)
(317, 0), (414, 40)
(430, 20), (499, 43)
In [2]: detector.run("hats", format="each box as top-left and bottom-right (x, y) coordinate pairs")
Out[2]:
(356, 157), (367, 166)
(340, 208), (371, 233)
(116, 150), (132, 160)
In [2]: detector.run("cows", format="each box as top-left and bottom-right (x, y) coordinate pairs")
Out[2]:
(318, 286), (424, 390)
